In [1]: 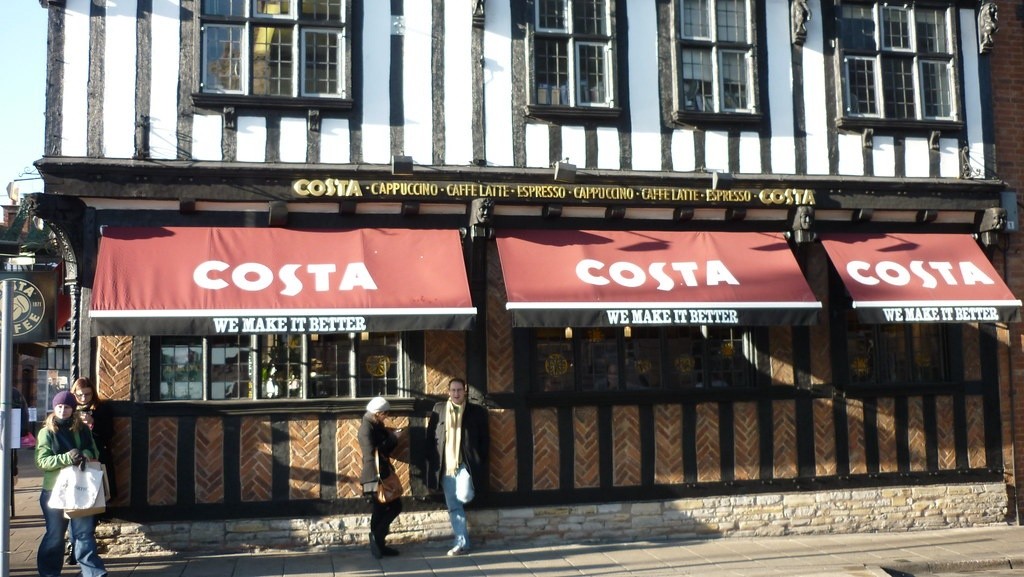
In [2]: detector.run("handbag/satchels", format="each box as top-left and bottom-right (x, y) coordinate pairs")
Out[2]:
(378, 472), (404, 503)
(47, 453), (111, 519)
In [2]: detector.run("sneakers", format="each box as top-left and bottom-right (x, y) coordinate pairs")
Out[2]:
(446, 544), (467, 555)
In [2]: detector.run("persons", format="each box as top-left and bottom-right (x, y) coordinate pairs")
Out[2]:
(476, 197), (495, 224)
(358, 397), (404, 559)
(33, 390), (109, 577)
(422, 378), (490, 556)
(993, 208), (1008, 230)
(11, 387), (30, 437)
(65, 377), (110, 565)
(799, 206), (815, 230)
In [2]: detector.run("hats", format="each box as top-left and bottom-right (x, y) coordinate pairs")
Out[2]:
(366, 396), (390, 412)
(51, 390), (78, 409)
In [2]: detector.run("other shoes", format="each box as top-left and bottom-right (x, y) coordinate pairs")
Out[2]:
(64, 544), (76, 564)
(369, 544), (399, 557)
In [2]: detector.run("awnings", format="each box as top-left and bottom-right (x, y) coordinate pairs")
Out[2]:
(86, 217), (479, 335)
(820, 231), (1023, 324)
(495, 224), (824, 330)
